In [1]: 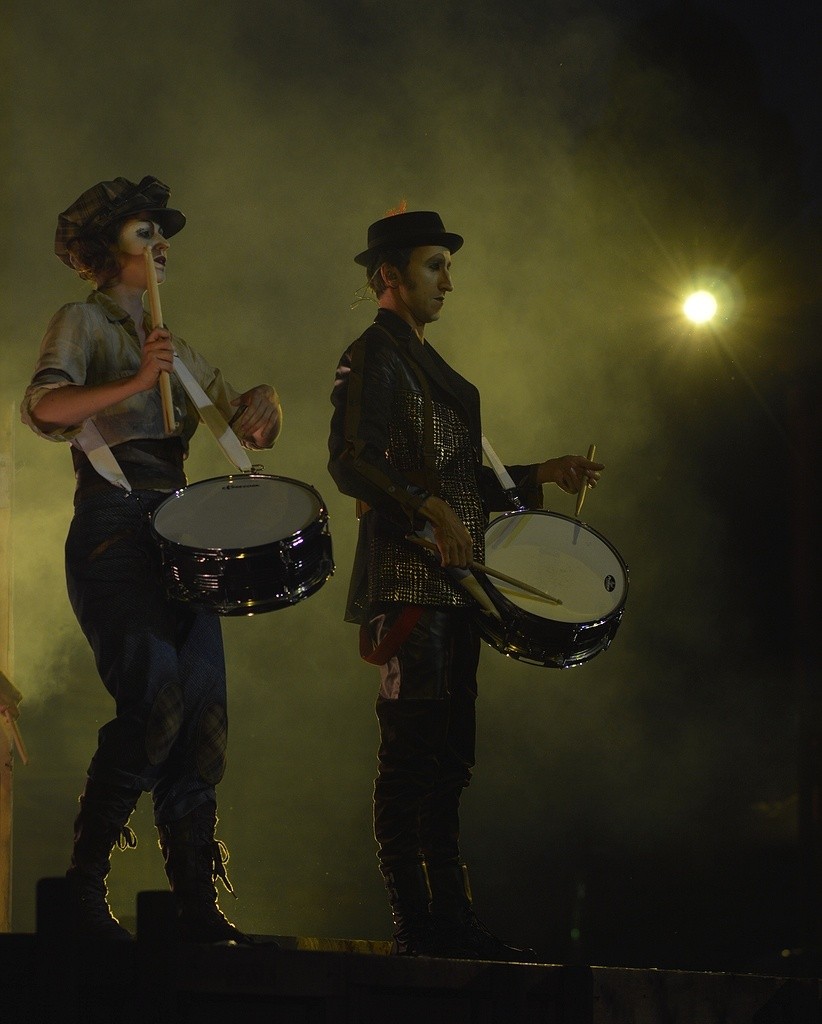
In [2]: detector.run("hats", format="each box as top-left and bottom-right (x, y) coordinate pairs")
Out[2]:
(354, 210), (465, 263)
(50, 173), (188, 278)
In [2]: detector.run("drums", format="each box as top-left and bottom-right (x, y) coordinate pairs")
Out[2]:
(476, 509), (631, 672)
(147, 470), (335, 618)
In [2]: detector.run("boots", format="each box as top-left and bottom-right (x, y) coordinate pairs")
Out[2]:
(50, 772), (139, 951)
(425, 856), (540, 962)
(373, 853), (441, 956)
(156, 810), (250, 942)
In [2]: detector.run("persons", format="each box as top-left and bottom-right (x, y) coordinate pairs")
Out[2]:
(323, 209), (605, 961)
(24, 173), (283, 931)
